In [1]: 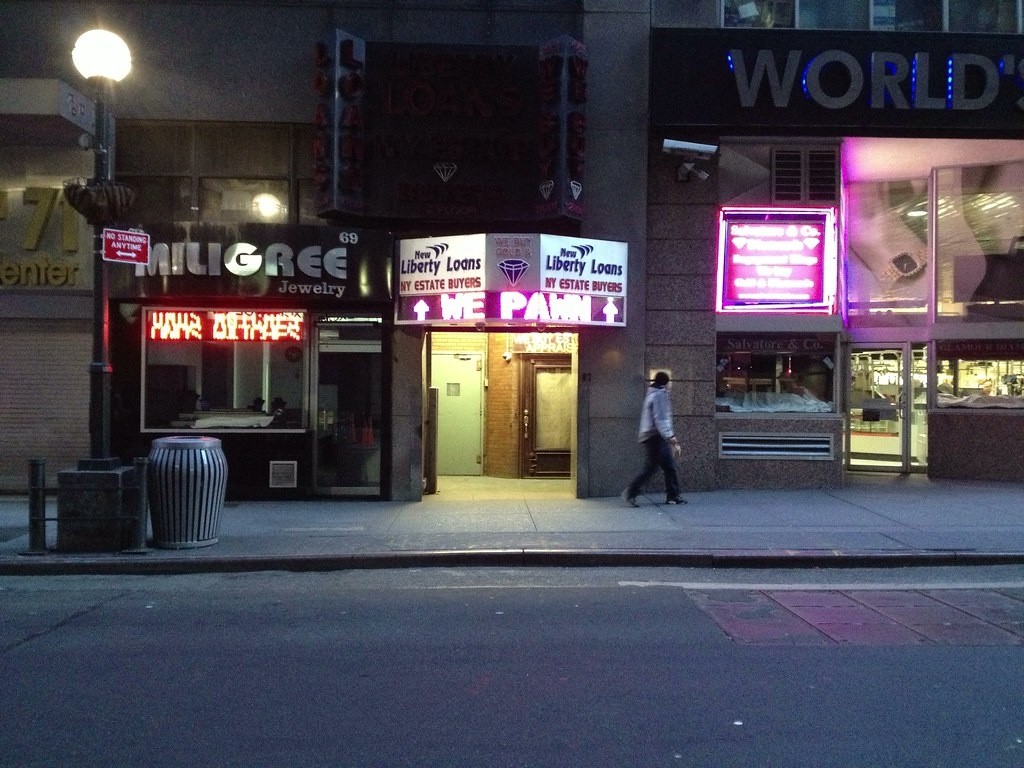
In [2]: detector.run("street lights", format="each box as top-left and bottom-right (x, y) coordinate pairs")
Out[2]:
(70, 27), (134, 471)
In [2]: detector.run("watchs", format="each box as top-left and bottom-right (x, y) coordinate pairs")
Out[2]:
(881, 248), (927, 293)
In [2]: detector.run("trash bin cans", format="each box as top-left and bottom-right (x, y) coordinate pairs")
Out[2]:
(145, 435), (228, 549)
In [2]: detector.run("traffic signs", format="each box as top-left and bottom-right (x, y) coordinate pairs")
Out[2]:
(100, 227), (155, 267)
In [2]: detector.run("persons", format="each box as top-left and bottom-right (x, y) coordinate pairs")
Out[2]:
(621, 372), (688, 506)
(979, 378), (993, 397)
(850, 181), (929, 299)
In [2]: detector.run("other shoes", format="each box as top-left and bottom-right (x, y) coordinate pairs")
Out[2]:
(621, 489), (640, 508)
(666, 494), (688, 504)
